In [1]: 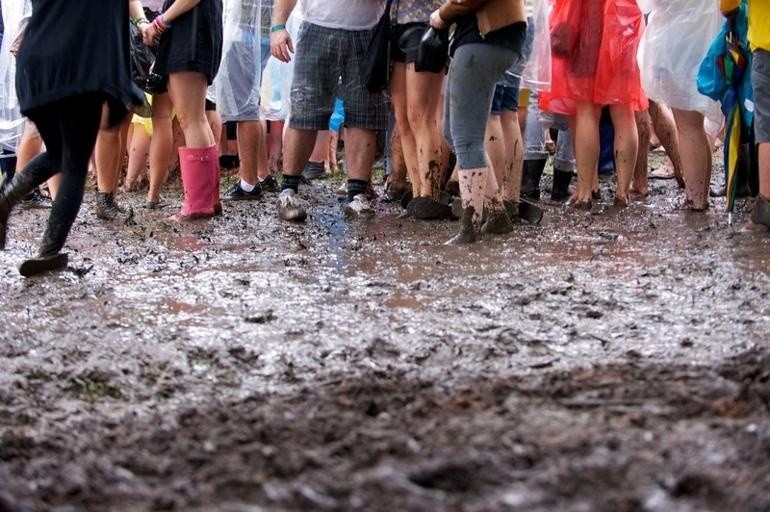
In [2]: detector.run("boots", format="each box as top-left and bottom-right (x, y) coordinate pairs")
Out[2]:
(0, 154), (88, 272)
(159, 142), (224, 230)
(520, 156), (574, 206)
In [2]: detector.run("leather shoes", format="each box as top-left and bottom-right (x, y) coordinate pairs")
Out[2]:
(223, 173), (277, 202)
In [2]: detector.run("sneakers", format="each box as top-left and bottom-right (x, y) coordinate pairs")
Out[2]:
(305, 164), (328, 187)
(96, 189), (138, 225)
(276, 185), (309, 225)
(345, 186), (379, 217)
